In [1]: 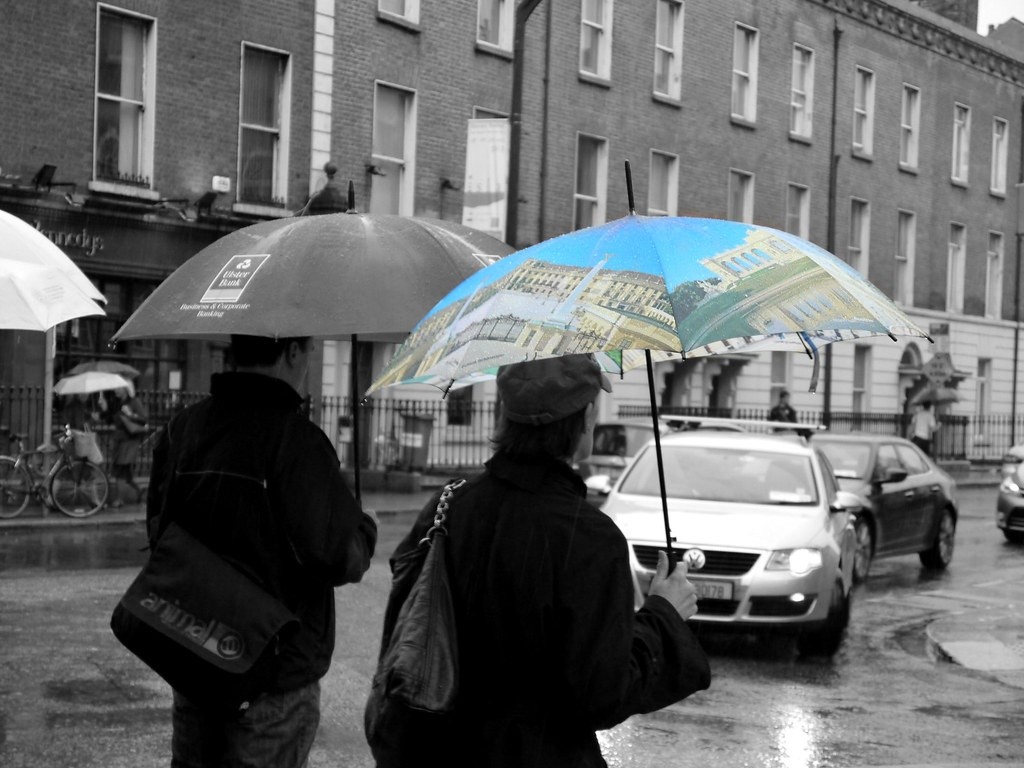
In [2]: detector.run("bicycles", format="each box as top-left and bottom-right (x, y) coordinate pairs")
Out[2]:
(0, 424), (109, 519)
(56, 420), (166, 512)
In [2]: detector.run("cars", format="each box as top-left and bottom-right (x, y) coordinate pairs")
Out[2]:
(580, 414), (750, 519)
(583, 428), (863, 656)
(785, 430), (959, 584)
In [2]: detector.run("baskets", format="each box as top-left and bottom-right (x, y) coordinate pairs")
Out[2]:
(58, 432), (96, 458)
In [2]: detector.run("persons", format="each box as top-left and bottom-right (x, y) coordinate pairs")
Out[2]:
(106, 334), (384, 768)
(364, 355), (715, 767)
(48, 389), (156, 504)
(911, 400), (942, 459)
(766, 392), (799, 434)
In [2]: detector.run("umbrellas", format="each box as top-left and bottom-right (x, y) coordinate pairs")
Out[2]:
(67, 359), (141, 382)
(113, 173), (510, 516)
(356, 158), (937, 580)
(49, 370), (141, 414)
(909, 380), (959, 418)
(0, 205), (107, 332)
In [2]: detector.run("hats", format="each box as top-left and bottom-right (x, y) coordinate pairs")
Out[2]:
(495, 351), (614, 426)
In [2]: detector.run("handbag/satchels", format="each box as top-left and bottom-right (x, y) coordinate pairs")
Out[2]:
(361, 480), (460, 760)
(83, 422), (103, 466)
(118, 411), (150, 433)
(110, 519), (301, 717)
(906, 413), (917, 441)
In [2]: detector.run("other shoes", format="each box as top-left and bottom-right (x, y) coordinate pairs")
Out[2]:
(136, 487), (147, 502)
(112, 499), (124, 508)
